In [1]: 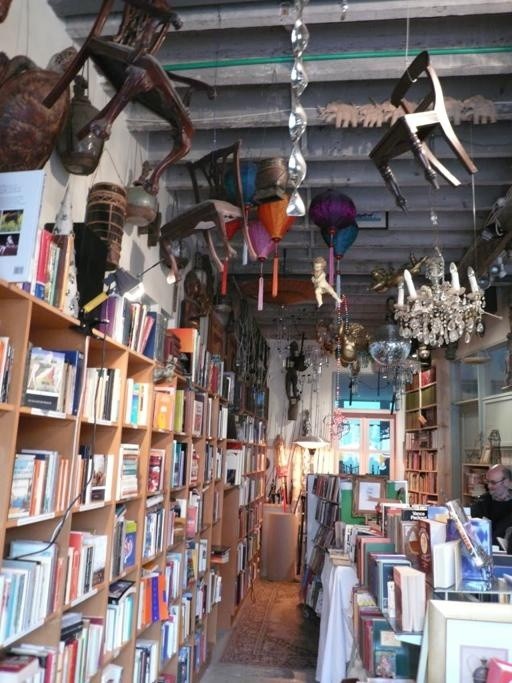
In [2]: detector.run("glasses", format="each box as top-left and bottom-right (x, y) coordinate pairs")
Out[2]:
(486, 479), (505, 484)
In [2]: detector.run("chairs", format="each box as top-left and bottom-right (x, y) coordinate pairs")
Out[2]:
(42, 0), (213, 185)
(369, 53), (477, 207)
(156, 139), (260, 276)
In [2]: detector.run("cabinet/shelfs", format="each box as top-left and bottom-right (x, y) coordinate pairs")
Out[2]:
(0, 234), (264, 683)
(401, 360), (489, 507)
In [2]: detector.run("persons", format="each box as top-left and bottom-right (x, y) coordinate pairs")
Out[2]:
(293, 470), (512, 683)
(471, 461), (512, 555)
(284, 340), (307, 406)
(311, 254), (342, 309)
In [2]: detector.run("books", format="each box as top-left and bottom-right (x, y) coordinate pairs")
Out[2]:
(405, 367), (437, 470)
(0, 170), (122, 518)
(102, 299), (265, 683)
(1, 532), (107, 683)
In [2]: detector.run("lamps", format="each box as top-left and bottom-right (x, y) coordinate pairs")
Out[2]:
(395, 249), (487, 349)
(295, 434), (329, 454)
